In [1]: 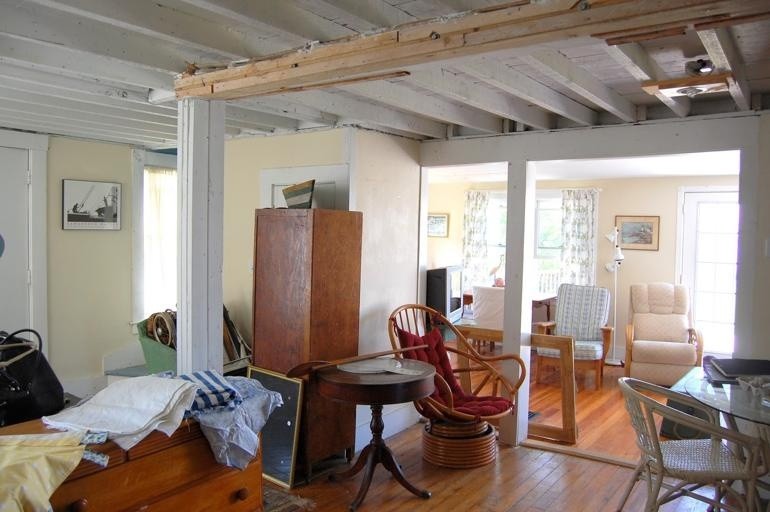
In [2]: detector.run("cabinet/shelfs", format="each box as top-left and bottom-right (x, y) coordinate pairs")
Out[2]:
(0, 416), (264, 512)
(253, 209), (362, 485)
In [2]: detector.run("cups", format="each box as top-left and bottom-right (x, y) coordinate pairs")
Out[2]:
(760, 383), (770, 408)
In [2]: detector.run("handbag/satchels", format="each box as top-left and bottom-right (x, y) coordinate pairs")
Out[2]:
(1, 328), (64, 427)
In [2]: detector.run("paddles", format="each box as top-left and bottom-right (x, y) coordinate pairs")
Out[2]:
(286, 344), (430, 379)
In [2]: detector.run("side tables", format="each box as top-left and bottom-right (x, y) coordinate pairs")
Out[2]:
(317, 357), (437, 512)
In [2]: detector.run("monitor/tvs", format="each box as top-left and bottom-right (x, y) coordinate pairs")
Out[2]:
(427, 266), (464, 327)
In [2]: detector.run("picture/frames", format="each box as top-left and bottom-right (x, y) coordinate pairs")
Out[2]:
(62, 178), (122, 231)
(428, 213), (449, 237)
(615, 215), (660, 251)
(246, 364), (305, 490)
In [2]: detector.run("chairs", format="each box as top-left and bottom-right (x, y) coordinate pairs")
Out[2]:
(533, 284), (614, 390)
(388, 303), (526, 469)
(617, 377), (770, 512)
(626, 284), (703, 387)
(472, 284), (503, 355)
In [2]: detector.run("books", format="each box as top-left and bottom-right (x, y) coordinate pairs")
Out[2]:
(703, 358), (770, 384)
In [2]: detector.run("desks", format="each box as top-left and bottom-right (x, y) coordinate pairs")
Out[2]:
(685, 368), (770, 512)
(472, 280), (558, 355)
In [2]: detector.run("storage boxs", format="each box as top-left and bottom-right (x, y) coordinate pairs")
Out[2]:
(659, 366), (720, 439)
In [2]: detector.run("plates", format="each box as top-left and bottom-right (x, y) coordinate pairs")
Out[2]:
(338, 358), (401, 375)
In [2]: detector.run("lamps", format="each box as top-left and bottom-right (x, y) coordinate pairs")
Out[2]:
(604, 225), (623, 365)
